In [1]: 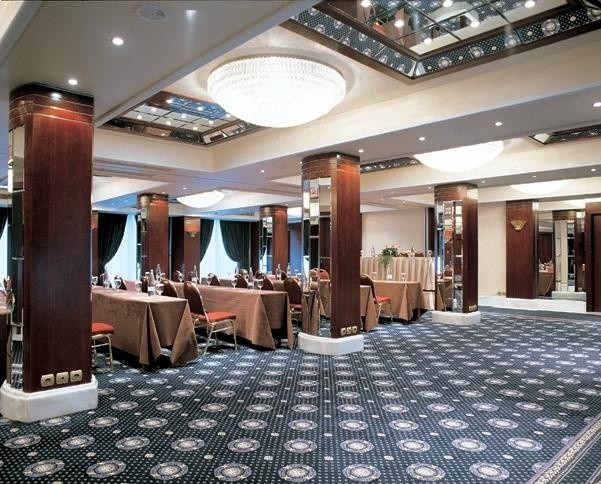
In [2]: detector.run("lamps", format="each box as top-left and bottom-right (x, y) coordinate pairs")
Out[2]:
(190, 46), (357, 130)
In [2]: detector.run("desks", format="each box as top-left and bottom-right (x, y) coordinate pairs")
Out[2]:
(372, 280), (428, 325)
(539, 269), (554, 296)
(360, 256), (440, 311)
(437, 276), (455, 310)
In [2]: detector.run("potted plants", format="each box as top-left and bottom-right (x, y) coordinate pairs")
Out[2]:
(378, 245), (398, 269)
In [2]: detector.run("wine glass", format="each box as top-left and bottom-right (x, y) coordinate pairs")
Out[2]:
(206, 276), (212, 288)
(135, 281), (142, 296)
(401, 272), (406, 283)
(115, 279), (121, 295)
(373, 272), (378, 282)
(156, 283), (164, 301)
(231, 278), (238, 290)
(257, 279), (264, 292)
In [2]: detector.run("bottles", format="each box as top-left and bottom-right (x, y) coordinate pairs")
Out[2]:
(103, 266), (109, 289)
(147, 269), (156, 299)
(192, 265), (198, 286)
(386, 260), (393, 281)
(247, 267), (254, 291)
(276, 262), (291, 280)
(156, 264), (161, 283)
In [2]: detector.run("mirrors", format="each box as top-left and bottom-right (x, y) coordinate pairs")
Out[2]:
(136, 207), (148, 281)
(6, 123), (27, 390)
(301, 175), (332, 338)
(258, 206), (272, 277)
(554, 219), (576, 292)
(435, 199), (464, 312)
